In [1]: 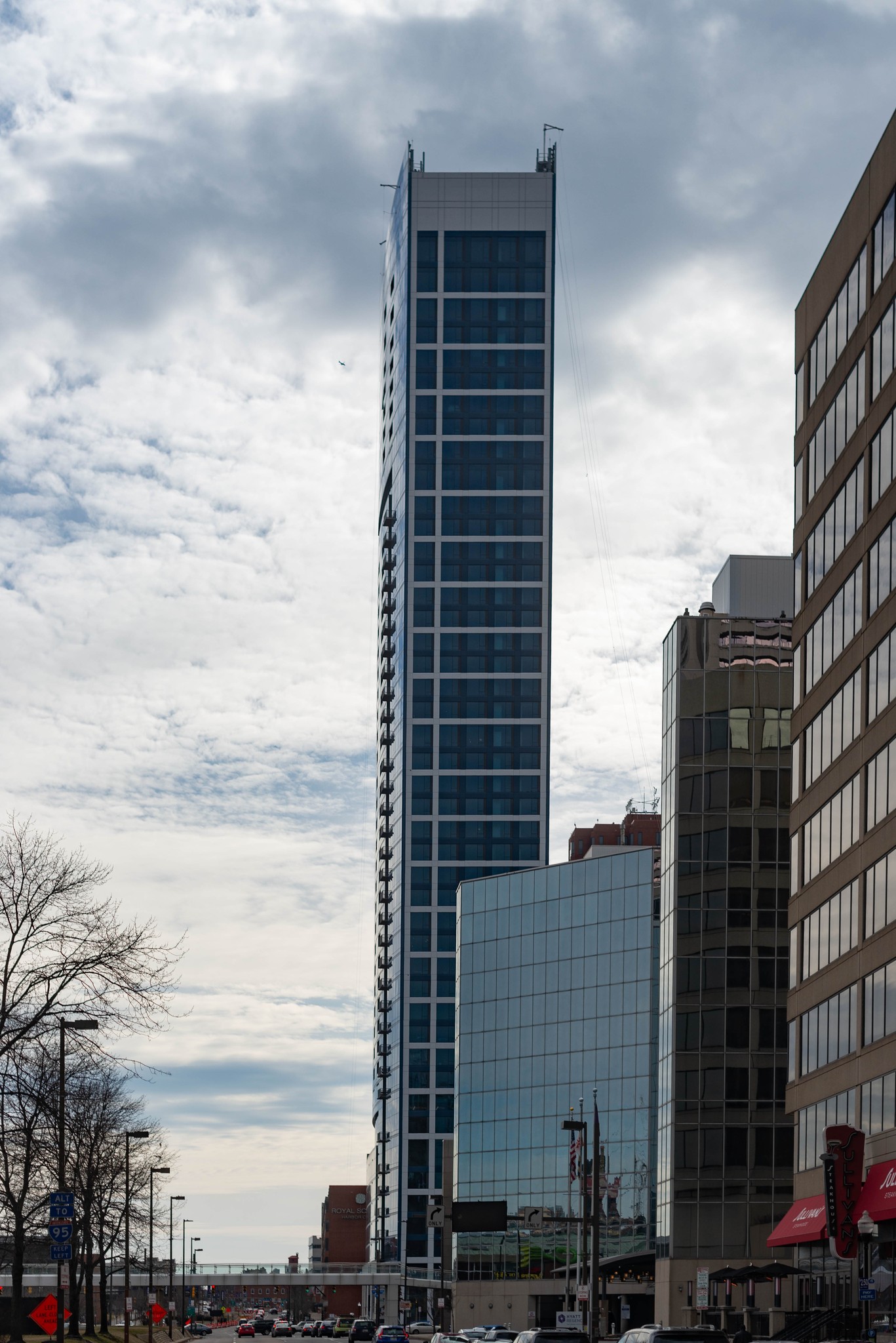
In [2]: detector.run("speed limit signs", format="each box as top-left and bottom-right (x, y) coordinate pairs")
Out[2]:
(49, 1220), (73, 1244)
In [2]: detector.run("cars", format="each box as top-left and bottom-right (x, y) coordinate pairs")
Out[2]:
(184, 1323), (212, 1336)
(423, 1325), (592, 1343)
(120, 1321), (135, 1326)
(270, 1317), (441, 1343)
(197, 1300), (278, 1338)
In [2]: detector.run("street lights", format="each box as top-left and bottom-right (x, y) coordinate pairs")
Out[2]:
(149, 1167), (194, 1343)
(125, 1131), (150, 1343)
(857, 1210), (874, 1343)
(57, 1017), (98, 1342)
(191, 1237), (203, 1274)
(560, 1120), (587, 1327)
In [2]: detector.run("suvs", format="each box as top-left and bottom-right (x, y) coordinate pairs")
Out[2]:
(617, 1324), (729, 1343)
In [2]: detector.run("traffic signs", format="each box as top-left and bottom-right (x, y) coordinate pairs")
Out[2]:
(425, 1205), (446, 1228)
(126, 1297), (132, 1312)
(525, 1206), (544, 1229)
(60, 1264), (70, 1289)
(149, 1293), (156, 1305)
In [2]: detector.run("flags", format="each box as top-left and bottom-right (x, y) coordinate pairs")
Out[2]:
(575, 1131), (584, 1181)
(569, 1130), (576, 1184)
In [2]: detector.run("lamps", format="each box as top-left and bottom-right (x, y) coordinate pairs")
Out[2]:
(507, 1303), (512, 1308)
(488, 1303), (493, 1309)
(679, 1286), (683, 1293)
(470, 1303), (474, 1309)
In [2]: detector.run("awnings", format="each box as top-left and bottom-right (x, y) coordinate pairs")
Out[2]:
(767, 1187), (863, 1248)
(853, 1158), (896, 1224)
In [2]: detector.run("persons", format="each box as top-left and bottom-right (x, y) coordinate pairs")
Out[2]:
(379, 1298), (385, 1319)
(732, 1324), (752, 1343)
(219, 1315), (223, 1328)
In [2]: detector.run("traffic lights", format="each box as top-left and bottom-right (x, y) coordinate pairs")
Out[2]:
(276, 1285), (336, 1293)
(211, 1285), (246, 1294)
